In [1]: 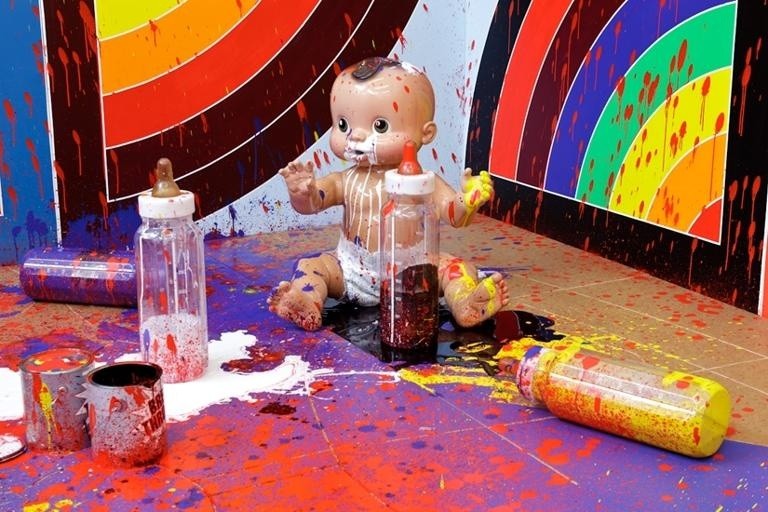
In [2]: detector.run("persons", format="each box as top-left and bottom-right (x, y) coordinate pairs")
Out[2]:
(268, 57), (511, 331)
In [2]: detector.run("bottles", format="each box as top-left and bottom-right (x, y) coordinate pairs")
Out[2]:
(377, 139), (440, 365)
(496, 345), (731, 458)
(131, 156), (211, 386)
(18, 244), (134, 308)
